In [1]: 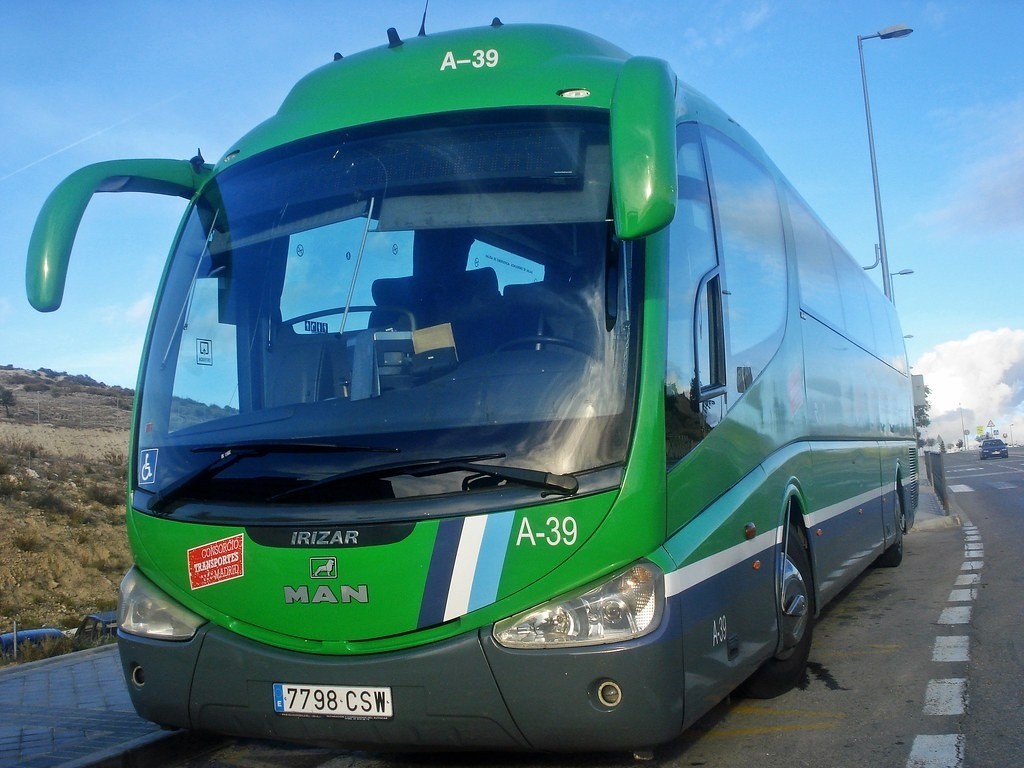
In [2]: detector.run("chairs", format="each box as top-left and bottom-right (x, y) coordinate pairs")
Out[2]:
(441, 263), (502, 352)
(368, 273), (436, 333)
(504, 272), (579, 358)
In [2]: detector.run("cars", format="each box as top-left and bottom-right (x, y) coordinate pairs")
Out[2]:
(978, 439), (1009, 460)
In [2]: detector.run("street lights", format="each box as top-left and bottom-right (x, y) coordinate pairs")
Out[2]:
(889, 268), (917, 307)
(856, 23), (917, 300)
(1009, 423), (1015, 448)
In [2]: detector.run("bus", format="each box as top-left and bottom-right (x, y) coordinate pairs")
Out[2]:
(22, 19), (925, 763)
